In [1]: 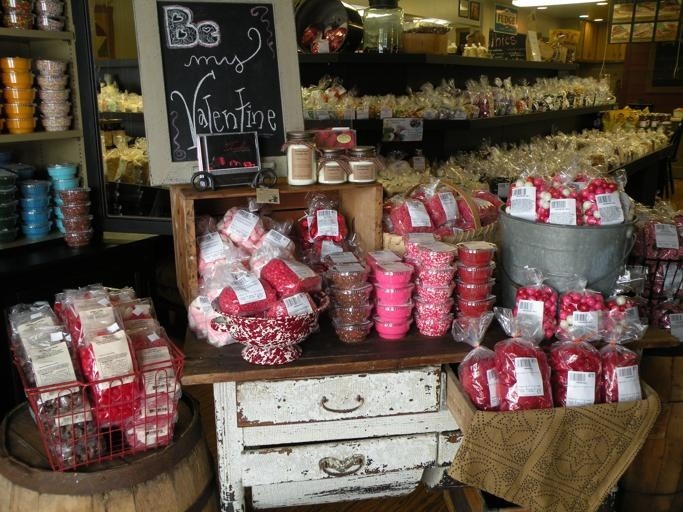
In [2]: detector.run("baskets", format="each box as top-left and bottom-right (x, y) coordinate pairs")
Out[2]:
(4, 319), (184, 473)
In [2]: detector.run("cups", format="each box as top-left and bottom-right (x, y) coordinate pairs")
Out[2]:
(639, 119), (660, 128)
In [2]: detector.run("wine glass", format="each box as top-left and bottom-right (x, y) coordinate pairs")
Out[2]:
(209, 291), (329, 365)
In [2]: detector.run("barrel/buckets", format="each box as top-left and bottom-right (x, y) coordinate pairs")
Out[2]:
(497, 204), (640, 308)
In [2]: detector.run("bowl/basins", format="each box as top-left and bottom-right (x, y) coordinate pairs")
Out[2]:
(0, 0), (94, 246)
(331, 240), (497, 344)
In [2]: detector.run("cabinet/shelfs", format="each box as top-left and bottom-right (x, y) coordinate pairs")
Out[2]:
(0, 0), (683, 512)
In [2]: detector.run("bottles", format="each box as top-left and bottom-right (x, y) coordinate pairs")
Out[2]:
(363, 0), (404, 53)
(285, 131), (377, 184)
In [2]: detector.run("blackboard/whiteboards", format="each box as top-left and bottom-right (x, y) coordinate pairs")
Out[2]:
(130, 0), (308, 188)
